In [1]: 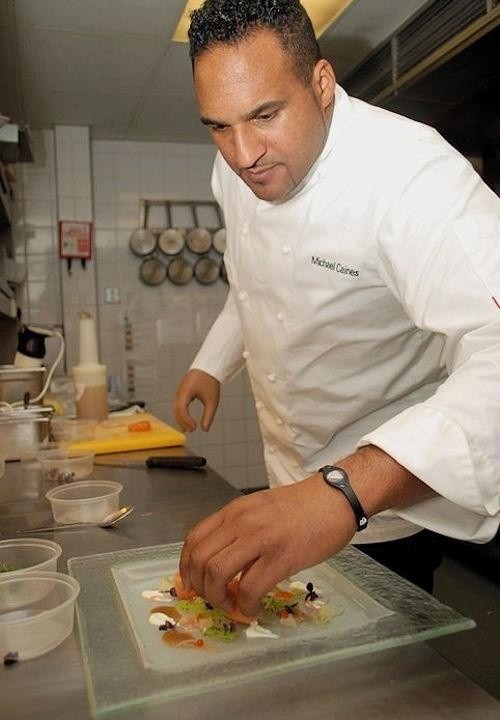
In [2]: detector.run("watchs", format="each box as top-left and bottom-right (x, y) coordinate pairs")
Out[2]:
(318, 463), (368, 531)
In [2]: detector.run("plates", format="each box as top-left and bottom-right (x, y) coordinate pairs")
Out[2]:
(68, 542), (479, 720)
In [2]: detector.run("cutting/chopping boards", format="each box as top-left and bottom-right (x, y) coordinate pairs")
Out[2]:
(67, 412), (187, 455)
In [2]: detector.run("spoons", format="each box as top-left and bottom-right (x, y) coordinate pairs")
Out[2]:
(15, 504), (135, 534)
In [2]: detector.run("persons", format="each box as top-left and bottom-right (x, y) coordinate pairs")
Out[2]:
(176, 1), (500, 624)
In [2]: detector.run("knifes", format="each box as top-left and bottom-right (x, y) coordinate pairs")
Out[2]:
(94, 456), (207, 469)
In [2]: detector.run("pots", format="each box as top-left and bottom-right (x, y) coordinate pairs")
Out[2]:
(130, 200), (228, 287)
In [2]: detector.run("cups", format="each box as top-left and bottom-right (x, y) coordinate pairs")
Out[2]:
(72, 364), (109, 420)
